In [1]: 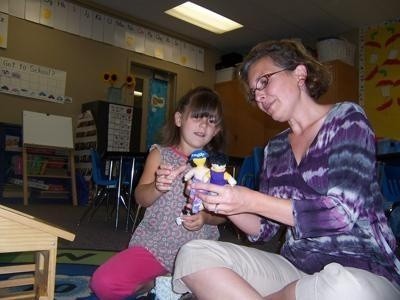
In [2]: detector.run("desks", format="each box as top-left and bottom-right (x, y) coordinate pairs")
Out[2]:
(103, 152), (149, 232)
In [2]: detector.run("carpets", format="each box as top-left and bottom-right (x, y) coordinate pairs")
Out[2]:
(1, 248), (156, 300)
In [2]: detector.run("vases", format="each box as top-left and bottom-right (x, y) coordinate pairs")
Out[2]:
(107, 87), (122, 104)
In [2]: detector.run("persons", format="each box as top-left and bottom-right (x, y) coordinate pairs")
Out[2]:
(174, 39), (400, 300)
(184, 149), (238, 214)
(90, 86), (227, 300)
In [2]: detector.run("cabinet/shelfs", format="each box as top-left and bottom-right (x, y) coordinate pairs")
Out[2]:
(212, 60), (358, 160)
(0, 121), (89, 206)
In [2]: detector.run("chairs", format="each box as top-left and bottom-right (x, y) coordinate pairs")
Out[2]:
(78, 149), (143, 227)
(225, 145), (264, 240)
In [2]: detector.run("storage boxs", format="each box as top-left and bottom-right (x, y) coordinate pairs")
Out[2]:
(215, 35), (357, 84)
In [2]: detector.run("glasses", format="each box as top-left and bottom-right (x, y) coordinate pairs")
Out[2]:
(248, 69), (288, 103)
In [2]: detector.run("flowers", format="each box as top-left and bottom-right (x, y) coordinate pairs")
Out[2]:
(104, 67), (136, 96)
